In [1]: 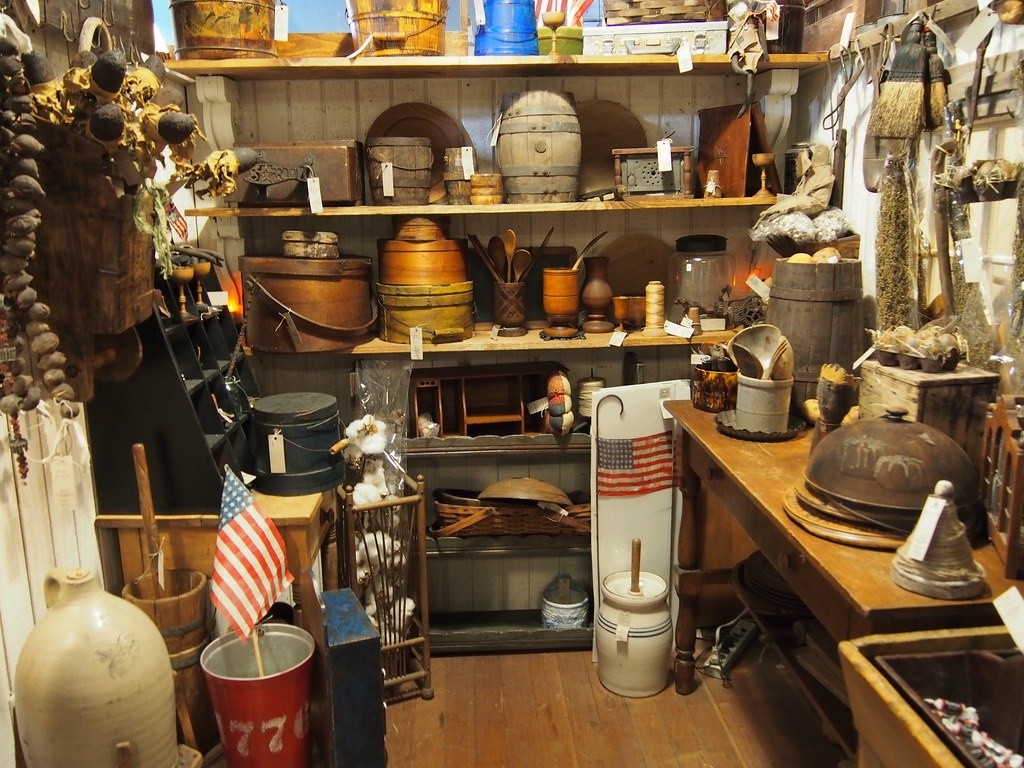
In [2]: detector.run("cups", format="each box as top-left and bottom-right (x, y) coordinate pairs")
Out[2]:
(471, 174), (502, 205)
(736, 372), (794, 433)
(493, 283), (526, 327)
(541, 586), (589, 630)
(445, 180), (471, 205)
(691, 365), (737, 413)
(443, 148), (479, 180)
(542, 268), (581, 338)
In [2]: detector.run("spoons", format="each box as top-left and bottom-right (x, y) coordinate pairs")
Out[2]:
(731, 342), (764, 380)
(487, 237), (505, 278)
(504, 230), (516, 282)
(514, 249), (531, 283)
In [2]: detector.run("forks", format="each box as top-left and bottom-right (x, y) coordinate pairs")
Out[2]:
(761, 340), (787, 379)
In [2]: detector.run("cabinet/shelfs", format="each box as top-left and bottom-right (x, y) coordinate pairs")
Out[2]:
(85, 259), (259, 516)
(153, 49), (831, 657)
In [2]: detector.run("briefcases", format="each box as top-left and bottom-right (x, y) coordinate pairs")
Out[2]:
(224, 138), (364, 205)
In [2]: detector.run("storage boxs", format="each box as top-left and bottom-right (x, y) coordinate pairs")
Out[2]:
(335, 470), (437, 702)
(229, 139), (361, 205)
(581, 20), (729, 57)
(838, 623), (1024, 768)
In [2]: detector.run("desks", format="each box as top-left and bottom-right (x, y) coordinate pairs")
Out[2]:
(95, 487), (342, 768)
(655, 393), (1024, 763)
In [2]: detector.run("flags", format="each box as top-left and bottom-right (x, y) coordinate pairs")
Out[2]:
(597, 431), (678, 496)
(211, 465), (295, 644)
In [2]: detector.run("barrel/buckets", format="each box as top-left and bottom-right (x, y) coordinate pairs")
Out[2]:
(121, 570), (206, 744)
(474, 0), (540, 55)
(171, 0), (280, 59)
(199, 623), (315, 768)
(366, 137), (432, 205)
(668, 235), (732, 324)
(345, 0), (449, 56)
(769, 257), (864, 413)
(496, 90), (582, 203)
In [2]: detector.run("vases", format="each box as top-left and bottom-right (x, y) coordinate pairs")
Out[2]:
(581, 256), (621, 333)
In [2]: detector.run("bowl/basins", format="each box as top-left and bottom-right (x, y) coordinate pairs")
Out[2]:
(612, 296), (646, 333)
